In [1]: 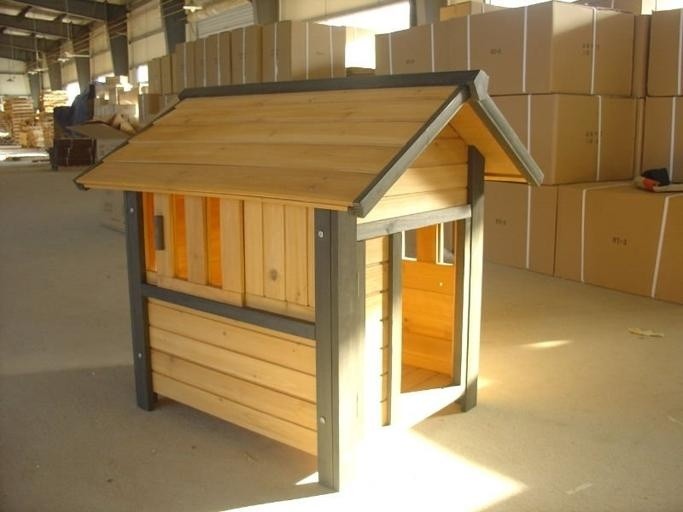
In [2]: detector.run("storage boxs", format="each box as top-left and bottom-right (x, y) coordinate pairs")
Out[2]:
(481, 2), (636, 94)
(650, 9), (683, 99)
(233, 26), (263, 85)
(376, 14), (470, 77)
(641, 95), (683, 183)
(265, 20), (347, 82)
(53, 50), (173, 171)
(173, 42), (195, 89)
(438, 3), (499, 18)
(486, 95), (637, 185)
(483, 180), (556, 278)
(555, 185), (681, 303)
(198, 31), (229, 88)
